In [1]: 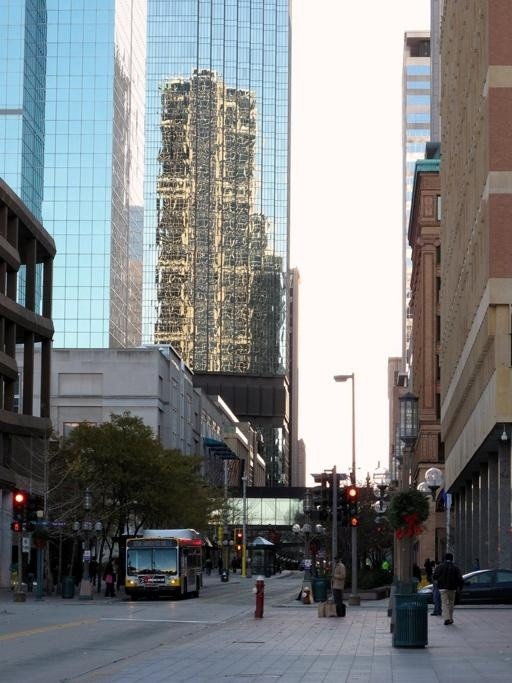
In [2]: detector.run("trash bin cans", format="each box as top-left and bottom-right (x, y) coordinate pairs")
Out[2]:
(264, 567), (270, 577)
(312, 577), (328, 602)
(221, 569), (228, 582)
(394, 594), (429, 648)
(61, 576), (74, 598)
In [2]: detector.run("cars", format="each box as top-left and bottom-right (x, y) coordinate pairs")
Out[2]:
(419, 569), (512, 605)
(297, 559), (312, 571)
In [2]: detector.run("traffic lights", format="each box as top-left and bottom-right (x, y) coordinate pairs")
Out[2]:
(345, 486), (359, 528)
(310, 472), (348, 524)
(12, 492), (26, 520)
(10, 522), (22, 533)
(234, 528), (243, 551)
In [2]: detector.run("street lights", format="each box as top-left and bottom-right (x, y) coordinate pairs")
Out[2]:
(73, 488), (102, 578)
(290, 488), (328, 557)
(334, 373), (357, 595)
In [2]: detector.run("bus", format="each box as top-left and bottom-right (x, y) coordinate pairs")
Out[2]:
(125, 528), (203, 599)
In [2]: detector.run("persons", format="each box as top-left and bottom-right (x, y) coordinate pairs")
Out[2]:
(204, 555), (213, 575)
(470, 556), (481, 572)
(231, 555), (238, 572)
(329, 555), (347, 617)
(430, 559), (444, 616)
(217, 556), (223, 575)
(88, 554), (99, 586)
(412, 561), (422, 584)
(381, 557), (389, 573)
(432, 552), (466, 625)
(102, 557), (117, 599)
(423, 555), (432, 584)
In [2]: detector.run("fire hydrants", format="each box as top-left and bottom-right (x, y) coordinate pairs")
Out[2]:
(252, 574), (265, 618)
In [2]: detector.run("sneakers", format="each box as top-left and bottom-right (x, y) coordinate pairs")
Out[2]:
(429, 612), (454, 626)
